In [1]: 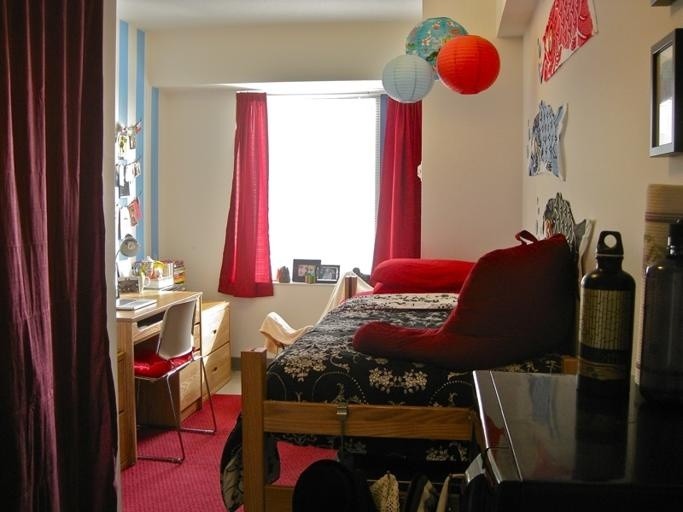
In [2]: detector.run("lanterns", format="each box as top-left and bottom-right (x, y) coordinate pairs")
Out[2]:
(382, 52), (433, 104)
(404, 16), (467, 81)
(435, 34), (499, 96)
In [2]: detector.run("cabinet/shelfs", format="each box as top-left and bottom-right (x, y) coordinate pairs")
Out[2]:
(465, 370), (683, 512)
(201, 300), (231, 404)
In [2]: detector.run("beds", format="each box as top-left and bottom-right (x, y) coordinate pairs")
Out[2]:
(242, 276), (582, 512)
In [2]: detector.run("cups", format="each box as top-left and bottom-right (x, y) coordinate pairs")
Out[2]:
(129, 274), (150, 293)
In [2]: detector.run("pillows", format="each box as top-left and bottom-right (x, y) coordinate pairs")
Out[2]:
(353, 229), (578, 373)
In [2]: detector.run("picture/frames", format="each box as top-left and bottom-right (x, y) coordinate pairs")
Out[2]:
(649, 26), (682, 156)
(291, 258), (340, 284)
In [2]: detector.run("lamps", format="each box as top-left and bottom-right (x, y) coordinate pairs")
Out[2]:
(115, 233), (141, 294)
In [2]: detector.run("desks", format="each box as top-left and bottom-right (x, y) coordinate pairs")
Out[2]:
(116, 291), (201, 469)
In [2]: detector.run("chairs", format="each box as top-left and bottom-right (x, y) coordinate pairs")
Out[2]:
(133, 297), (217, 465)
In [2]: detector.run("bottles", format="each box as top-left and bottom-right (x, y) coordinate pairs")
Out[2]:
(276, 266), (290, 283)
(576, 219), (683, 403)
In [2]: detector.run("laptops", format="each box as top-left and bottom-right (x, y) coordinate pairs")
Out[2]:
(115, 261), (158, 311)
